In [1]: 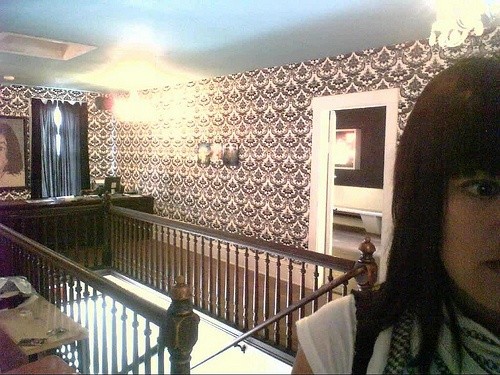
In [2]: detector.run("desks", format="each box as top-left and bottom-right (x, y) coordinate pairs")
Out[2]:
(0, 293), (90, 375)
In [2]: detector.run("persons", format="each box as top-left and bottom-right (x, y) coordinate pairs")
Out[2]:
(290, 58), (500, 375)
(1, 118), (25, 186)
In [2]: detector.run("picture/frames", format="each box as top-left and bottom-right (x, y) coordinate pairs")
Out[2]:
(0, 115), (28, 190)
